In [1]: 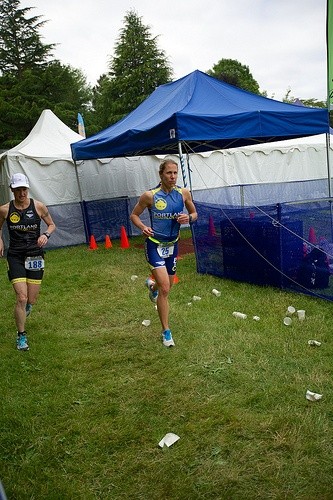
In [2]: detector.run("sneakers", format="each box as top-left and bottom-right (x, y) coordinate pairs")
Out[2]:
(16, 332), (30, 351)
(25, 303), (35, 318)
(145, 280), (160, 303)
(160, 328), (176, 347)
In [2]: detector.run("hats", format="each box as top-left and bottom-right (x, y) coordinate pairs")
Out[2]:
(9, 173), (31, 190)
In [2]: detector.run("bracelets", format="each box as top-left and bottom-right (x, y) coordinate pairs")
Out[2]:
(188, 213), (192, 223)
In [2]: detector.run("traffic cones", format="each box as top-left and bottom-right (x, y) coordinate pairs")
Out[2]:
(303, 227), (331, 266)
(118, 226), (130, 249)
(209, 215), (216, 236)
(167, 272), (178, 283)
(105, 235), (112, 249)
(89, 235), (97, 249)
(250, 212), (254, 221)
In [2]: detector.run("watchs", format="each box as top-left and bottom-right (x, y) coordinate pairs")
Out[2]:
(43, 231), (50, 238)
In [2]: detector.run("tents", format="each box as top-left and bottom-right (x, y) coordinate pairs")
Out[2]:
(71, 69), (332, 267)
(0, 108), (333, 252)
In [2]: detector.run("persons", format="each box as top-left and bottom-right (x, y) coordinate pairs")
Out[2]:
(129, 159), (197, 347)
(0, 173), (56, 349)
